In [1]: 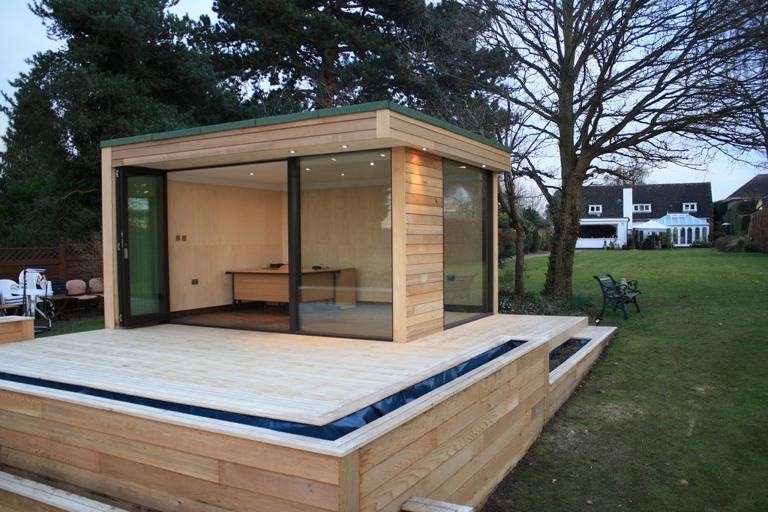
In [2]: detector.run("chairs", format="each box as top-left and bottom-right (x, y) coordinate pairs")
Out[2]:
(0, 267), (104, 345)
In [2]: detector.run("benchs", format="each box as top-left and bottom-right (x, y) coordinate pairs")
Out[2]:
(592, 273), (642, 320)
(444, 271), (478, 313)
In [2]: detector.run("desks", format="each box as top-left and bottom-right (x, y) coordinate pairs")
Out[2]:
(225, 267), (358, 312)
